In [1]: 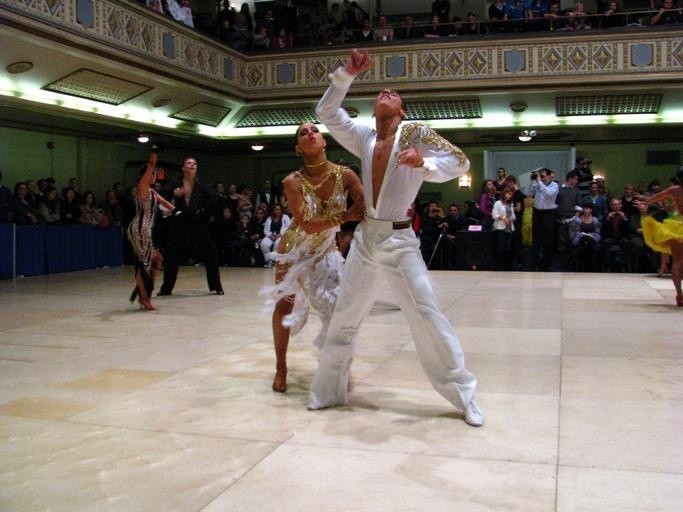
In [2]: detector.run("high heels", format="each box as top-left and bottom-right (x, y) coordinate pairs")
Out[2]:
(137, 297), (156, 312)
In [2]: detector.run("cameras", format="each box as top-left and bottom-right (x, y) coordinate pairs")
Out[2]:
(585, 159), (592, 165)
(531, 171), (541, 180)
(432, 208), (441, 212)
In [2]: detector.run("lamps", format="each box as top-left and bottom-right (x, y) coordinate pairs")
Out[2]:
(137, 134), (148, 144)
(458, 170), (470, 190)
(516, 129), (534, 144)
(250, 142), (265, 151)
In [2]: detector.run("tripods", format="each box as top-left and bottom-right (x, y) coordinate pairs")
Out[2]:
(427, 232), (456, 270)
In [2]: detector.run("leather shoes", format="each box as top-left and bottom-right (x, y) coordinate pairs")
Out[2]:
(157, 289), (172, 296)
(306, 400), (330, 410)
(216, 287), (224, 295)
(270, 365), (288, 393)
(462, 398), (485, 427)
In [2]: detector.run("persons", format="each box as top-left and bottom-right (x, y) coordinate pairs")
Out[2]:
(307, 48), (485, 426)
(259, 123), (416, 392)
